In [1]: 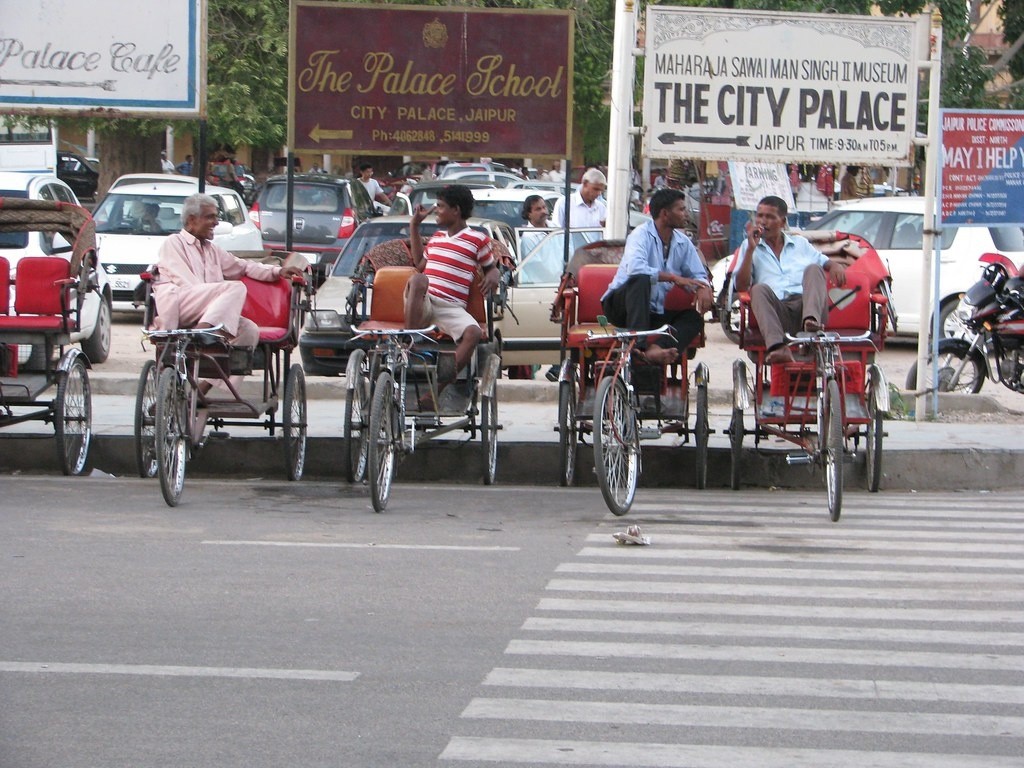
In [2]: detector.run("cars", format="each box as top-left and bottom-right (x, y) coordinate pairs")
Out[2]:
(0, 108), (1024, 387)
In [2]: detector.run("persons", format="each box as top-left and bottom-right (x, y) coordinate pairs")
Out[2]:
(129, 203), (161, 234)
(151, 193), (303, 356)
(734, 197), (846, 363)
(159, 148), (325, 207)
(520, 195), (559, 265)
(631, 157), (923, 205)
(401, 159), (565, 191)
(355, 161), (392, 215)
(600, 188), (714, 364)
(386, 186), (501, 412)
(552, 167), (605, 233)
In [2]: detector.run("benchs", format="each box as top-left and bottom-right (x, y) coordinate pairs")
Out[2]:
(0, 257), (78, 338)
(354, 266), (492, 344)
(563, 264), (703, 348)
(738, 270), (888, 349)
(222, 275), (292, 343)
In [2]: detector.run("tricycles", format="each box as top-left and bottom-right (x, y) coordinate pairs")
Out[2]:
(719, 227), (900, 522)
(0, 195), (106, 477)
(340, 234), (521, 514)
(546, 236), (717, 518)
(131, 259), (308, 510)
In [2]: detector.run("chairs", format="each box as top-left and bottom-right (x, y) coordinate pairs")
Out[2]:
(130, 202), (183, 231)
(891, 222), (925, 248)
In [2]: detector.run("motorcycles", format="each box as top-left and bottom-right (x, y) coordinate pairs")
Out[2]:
(903, 253), (1024, 403)
